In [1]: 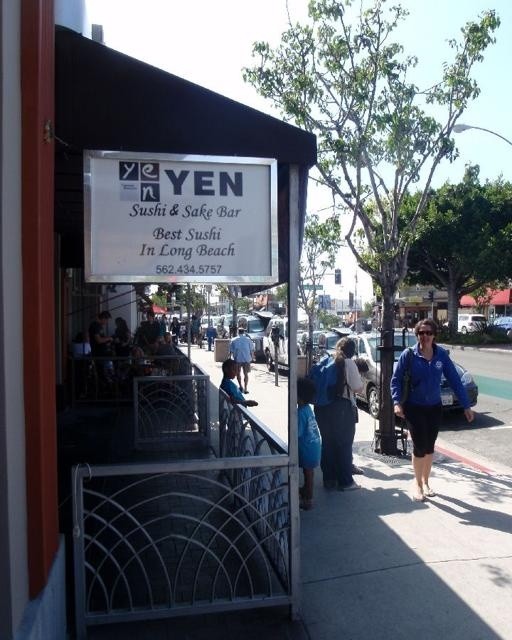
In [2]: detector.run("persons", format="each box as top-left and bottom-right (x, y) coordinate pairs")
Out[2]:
(216, 357), (259, 486)
(346, 358), (369, 477)
(389, 318), (474, 503)
(229, 327), (254, 393)
(295, 376), (323, 512)
(66, 302), (221, 388)
(311, 336), (365, 492)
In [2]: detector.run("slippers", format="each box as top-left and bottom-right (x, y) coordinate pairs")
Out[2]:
(422, 481), (437, 499)
(413, 483), (426, 502)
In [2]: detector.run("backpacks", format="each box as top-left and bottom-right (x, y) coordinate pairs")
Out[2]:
(306, 351), (346, 408)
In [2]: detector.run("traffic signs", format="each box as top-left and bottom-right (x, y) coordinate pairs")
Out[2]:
(299, 284), (324, 290)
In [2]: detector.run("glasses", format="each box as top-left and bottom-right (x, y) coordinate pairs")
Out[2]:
(417, 329), (434, 338)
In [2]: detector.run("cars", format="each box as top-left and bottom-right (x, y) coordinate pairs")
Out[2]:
(486, 316), (512, 340)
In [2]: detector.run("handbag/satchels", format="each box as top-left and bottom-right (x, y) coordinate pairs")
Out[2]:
(398, 347), (412, 407)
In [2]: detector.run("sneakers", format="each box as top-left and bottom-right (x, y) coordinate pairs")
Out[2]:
(351, 464), (366, 475)
(239, 386), (249, 395)
(322, 480), (361, 492)
(298, 487), (314, 511)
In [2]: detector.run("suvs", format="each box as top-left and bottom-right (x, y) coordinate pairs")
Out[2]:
(439, 314), (487, 334)
